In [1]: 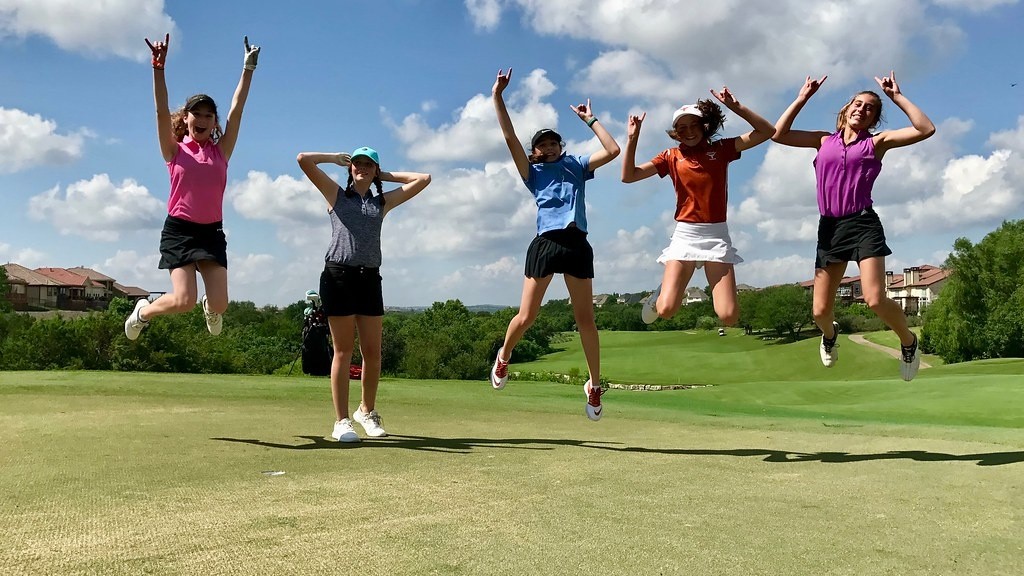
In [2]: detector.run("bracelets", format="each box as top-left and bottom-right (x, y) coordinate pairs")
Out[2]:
(153, 67), (164, 70)
(588, 117), (598, 127)
(152, 60), (164, 67)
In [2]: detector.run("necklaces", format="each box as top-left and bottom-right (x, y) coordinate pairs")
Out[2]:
(361, 197), (369, 214)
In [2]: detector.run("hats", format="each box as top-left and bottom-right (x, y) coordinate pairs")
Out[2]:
(184, 95), (217, 113)
(349, 147), (379, 165)
(673, 105), (704, 127)
(531, 129), (562, 146)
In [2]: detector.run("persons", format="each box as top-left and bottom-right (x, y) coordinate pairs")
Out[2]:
(297, 147), (431, 442)
(490, 68), (620, 421)
(125, 35), (260, 341)
(622, 85), (777, 325)
(771, 71), (936, 381)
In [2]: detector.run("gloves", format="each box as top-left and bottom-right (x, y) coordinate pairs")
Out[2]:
(243, 35), (260, 71)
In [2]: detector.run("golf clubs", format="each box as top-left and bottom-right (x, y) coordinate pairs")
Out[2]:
(308, 309), (325, 326)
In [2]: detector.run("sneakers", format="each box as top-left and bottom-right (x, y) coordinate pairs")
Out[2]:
(353, 404), (386, 437)
(641, 283), (659, 324)
(901, 329), (920, 381)
(491, 347), (512, 389)
(124, 297), (151, 341)
(202, 294), (222, 335)
(584, 379), (608, 421)
(332, 417), (359, 442)
(820, 321), (842, 368)
